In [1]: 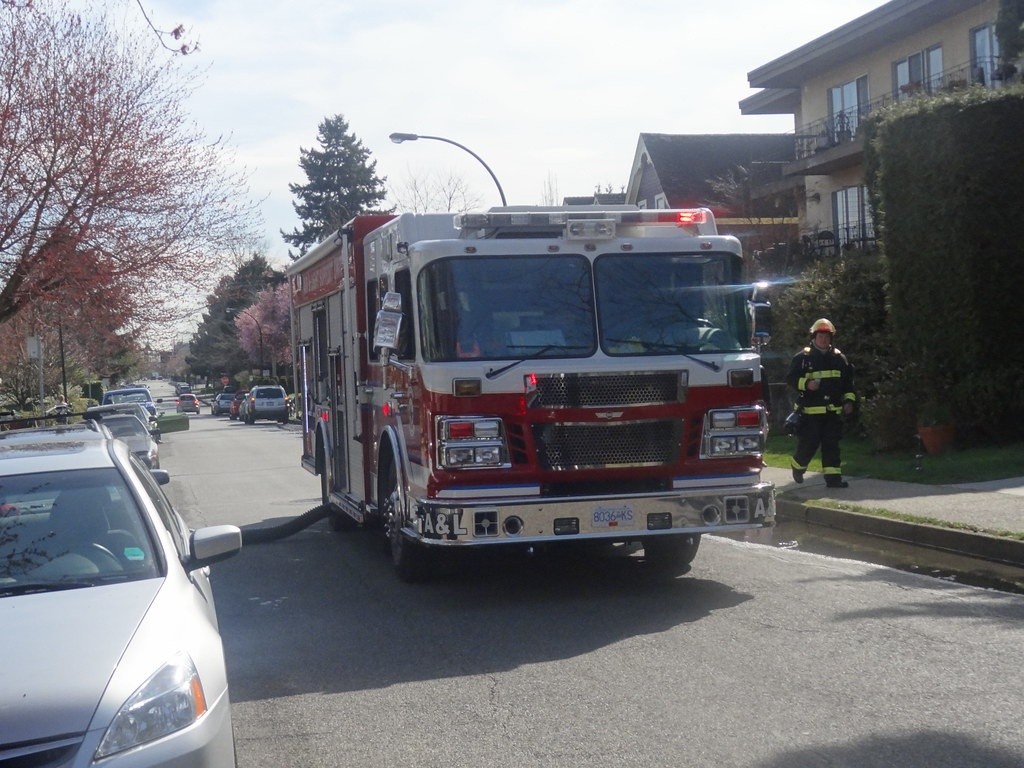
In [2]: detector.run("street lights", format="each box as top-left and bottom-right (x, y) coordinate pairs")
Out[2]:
(226, 307), (263, 377)
(389, 133), (507, 207)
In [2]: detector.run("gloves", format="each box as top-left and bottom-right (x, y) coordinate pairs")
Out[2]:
(844, 402), (852, 416)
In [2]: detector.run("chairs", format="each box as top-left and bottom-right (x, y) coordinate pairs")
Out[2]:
(31, 492), (139, 568)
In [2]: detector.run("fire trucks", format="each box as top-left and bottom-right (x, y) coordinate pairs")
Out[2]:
(285, 206), (778, 584)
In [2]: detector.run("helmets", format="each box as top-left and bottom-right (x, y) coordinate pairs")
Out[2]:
(809, 318), (837, 336)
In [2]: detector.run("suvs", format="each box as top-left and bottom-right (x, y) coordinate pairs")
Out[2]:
(86, 384), (158, 441)
(175, 382), (191, 396)
(210, 393), (236, 416)
(177, 394), (200, 414)
(0, 412), (244, 768)
(230, 386), (289, 425)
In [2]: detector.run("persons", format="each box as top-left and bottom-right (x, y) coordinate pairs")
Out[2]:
(55, 395), (69, 425)
(788, 319), (858, 488)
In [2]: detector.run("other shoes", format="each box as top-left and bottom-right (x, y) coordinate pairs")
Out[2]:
(791, 464), (804, 483)
(826, 479), (849, 488)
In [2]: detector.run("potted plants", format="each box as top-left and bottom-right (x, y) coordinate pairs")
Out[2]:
(915, 407), (956, 454)
(834, 110), (853, 143)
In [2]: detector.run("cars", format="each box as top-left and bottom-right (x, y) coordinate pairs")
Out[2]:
(100, 414), (161, 470)
